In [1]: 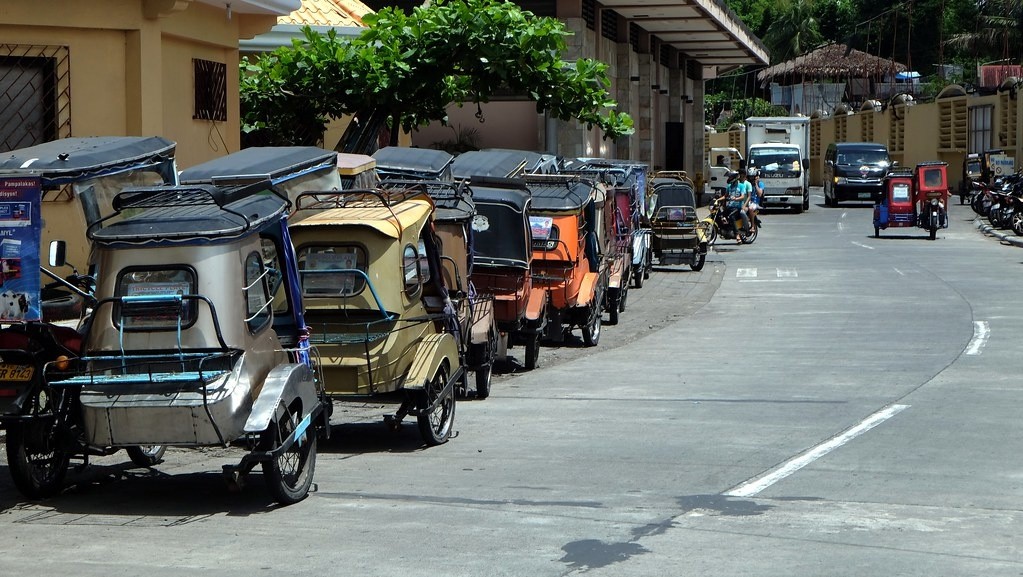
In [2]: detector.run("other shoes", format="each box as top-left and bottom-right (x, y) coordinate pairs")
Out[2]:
(736, 235), (741, 243)
(750, 226), (756, 232)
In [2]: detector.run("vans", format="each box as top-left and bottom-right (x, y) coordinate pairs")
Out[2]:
(823, 142), (899, 206)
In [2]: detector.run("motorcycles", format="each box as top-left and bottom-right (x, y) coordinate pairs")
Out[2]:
(970, 168), (1023, 236)
(960, 153), (1006, 204)
(698, 194), (762, 246)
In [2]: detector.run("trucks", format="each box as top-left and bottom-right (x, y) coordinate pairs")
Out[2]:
(708, 116), (811, 213)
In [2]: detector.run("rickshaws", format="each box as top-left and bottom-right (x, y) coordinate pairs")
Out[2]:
(173, 146), (647, 445)
(873, 161), (952, 239)
(646, 171), (709, 271)
(0, 135), (329, 505)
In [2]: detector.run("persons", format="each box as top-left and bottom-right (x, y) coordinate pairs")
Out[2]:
(542, 221), (550, 228)
(737, 168), (752, 237)
(713, 169), (742, 245)
(346, 260), (352, 269)
(177, 290), (183, 294)
(715, 155), (728, 168)
(746, 166), (765, 232)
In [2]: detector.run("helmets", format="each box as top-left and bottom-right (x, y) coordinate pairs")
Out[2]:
(724, 170), (739, 186)
(747, 167), (760, 180)
